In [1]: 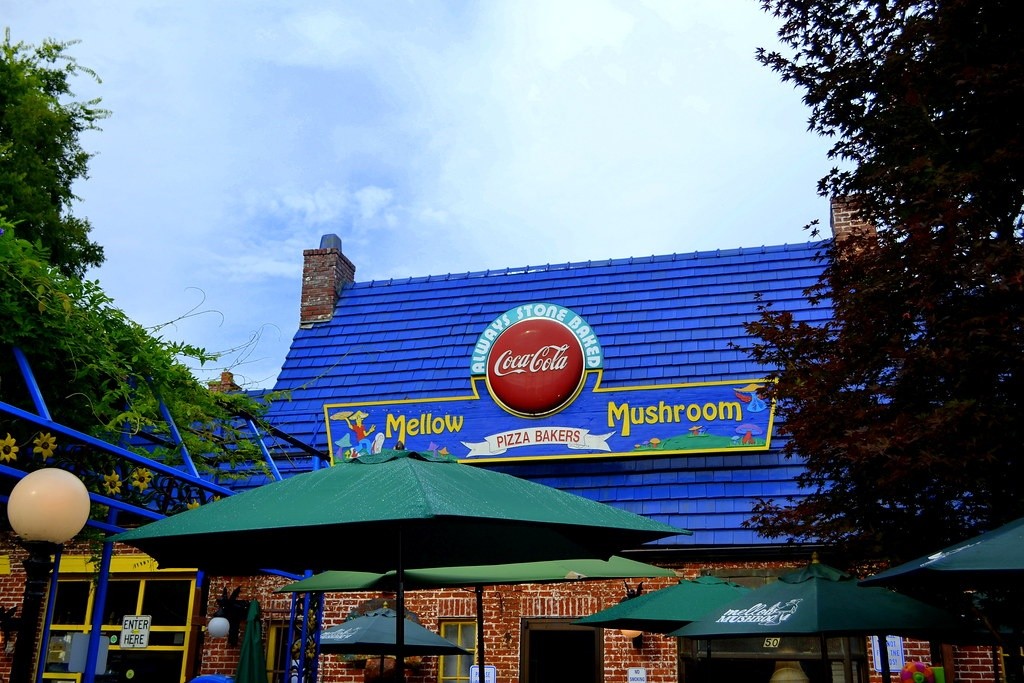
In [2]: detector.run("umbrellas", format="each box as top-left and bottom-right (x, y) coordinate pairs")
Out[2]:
(319, 601), (474, 683)
(273, 554), (677, 683)
(666, 517), (1024, 683)
(232, 597), (267, 683)
(569, 572), (756, 683)
(94, 440), (694, 683)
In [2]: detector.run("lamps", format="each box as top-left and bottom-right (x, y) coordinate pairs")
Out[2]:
(207, 606), (230, 638)
(619, 591), (641, 639)
